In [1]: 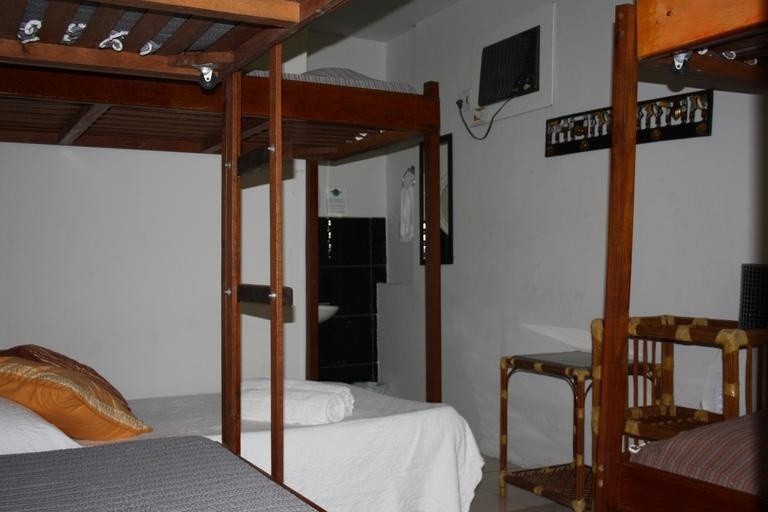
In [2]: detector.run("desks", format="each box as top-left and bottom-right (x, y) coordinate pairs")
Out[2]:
(499, 349), (663, 512)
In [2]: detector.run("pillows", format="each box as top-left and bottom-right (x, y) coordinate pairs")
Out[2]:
(1, 343), (152, 442)
(1, 397), (84, 455)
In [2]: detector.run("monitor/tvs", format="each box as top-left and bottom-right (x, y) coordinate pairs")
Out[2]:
(477, 24), (541, 106)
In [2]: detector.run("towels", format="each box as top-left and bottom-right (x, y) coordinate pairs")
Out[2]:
(237, 388), (347, 426)
(399, 184), (413, 244)
(237, 380), (355, 417)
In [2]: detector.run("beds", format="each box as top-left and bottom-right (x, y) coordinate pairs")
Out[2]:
(0, 0), (484, 511)
(591, 0), (768, 512)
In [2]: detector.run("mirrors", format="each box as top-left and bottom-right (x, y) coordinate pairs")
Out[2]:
(419, 133), (453, 266)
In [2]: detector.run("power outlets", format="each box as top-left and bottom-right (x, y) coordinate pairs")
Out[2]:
(456, 89), (472, 112)
